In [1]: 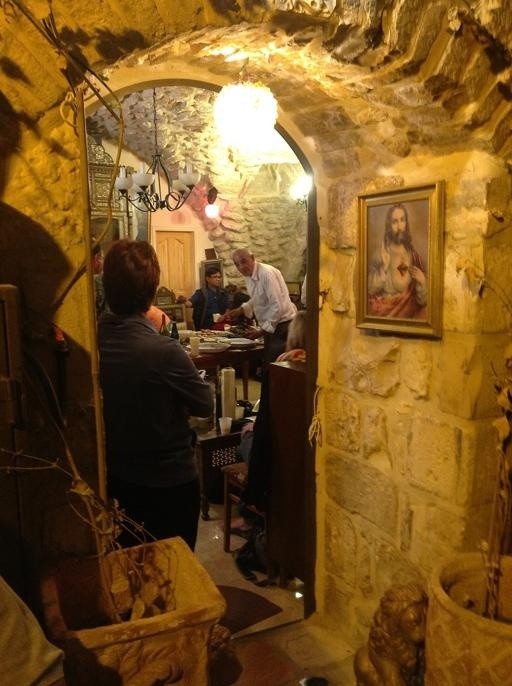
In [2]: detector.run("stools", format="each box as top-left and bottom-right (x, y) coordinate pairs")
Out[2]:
(223, 461), (267, 550)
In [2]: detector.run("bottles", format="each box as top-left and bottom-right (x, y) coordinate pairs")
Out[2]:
(159, 314), (168, 335)
(170, 315), (179, 341)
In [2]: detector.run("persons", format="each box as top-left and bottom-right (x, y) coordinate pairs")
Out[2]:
(217, 247), (298, 406)
(224, 283), (254, 320)
(177, 266), (231, 331)
(368, 203), (427, 319)
(93, 245), (173, 335)
(217, 308), (306, 588)
(96, 237), (216, 562)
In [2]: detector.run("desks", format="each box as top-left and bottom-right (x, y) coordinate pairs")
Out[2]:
(196, 427), (242, 520)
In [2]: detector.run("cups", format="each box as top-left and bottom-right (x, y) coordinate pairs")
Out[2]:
(212, 313), (220, 323)
(234, 406), (244, 420)
(218, 416), (232, 434)
(189, 337), (200, 356)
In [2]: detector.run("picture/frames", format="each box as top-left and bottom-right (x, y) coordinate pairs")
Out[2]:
(354, 180), (447, 339)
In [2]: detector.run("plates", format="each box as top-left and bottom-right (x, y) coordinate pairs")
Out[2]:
(193, 328), (233, 342)
(216, 337), (256, 349)
(169, 329), (198, 335)
(186, 342), (231, 354)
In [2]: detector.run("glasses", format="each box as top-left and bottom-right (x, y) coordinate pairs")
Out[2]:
(209, 275), (221, 278)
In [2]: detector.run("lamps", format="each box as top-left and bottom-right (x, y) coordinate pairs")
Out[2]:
(115, 86), (201, 213)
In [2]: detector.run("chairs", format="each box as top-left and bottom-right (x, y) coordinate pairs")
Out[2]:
(154, 287), (187, 330)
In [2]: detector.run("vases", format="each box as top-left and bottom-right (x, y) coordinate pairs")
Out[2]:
(36, 535), (227, 686)
(424, 551), (512, 686)
